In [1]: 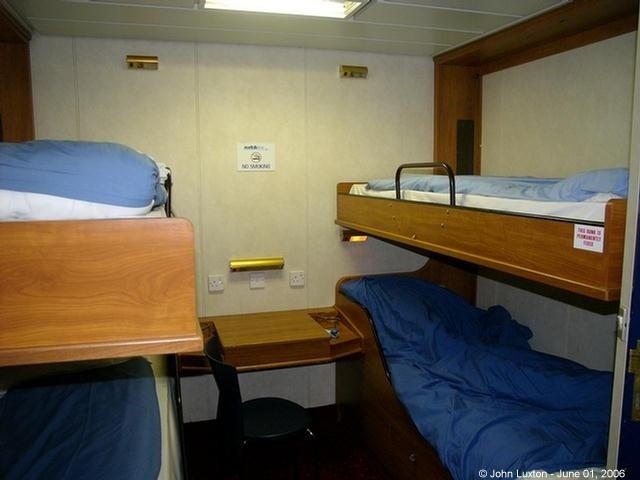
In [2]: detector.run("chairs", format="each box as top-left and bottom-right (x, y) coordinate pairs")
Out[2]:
(201, 322), (331, 478)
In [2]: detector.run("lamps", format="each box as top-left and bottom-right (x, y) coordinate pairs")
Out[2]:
(125, 53), (160, 71)
(227, 257), (285, 274)
(338, 63), (370, 80)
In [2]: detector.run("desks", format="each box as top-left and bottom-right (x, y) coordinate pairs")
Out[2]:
(166, 298), (370, 479)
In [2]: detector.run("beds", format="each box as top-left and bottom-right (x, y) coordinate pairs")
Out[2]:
(0, 134), (207, 372)
(328, 258), (638, 479)
(1, 351), (183, 479)
(334, 160), (631, 312)
